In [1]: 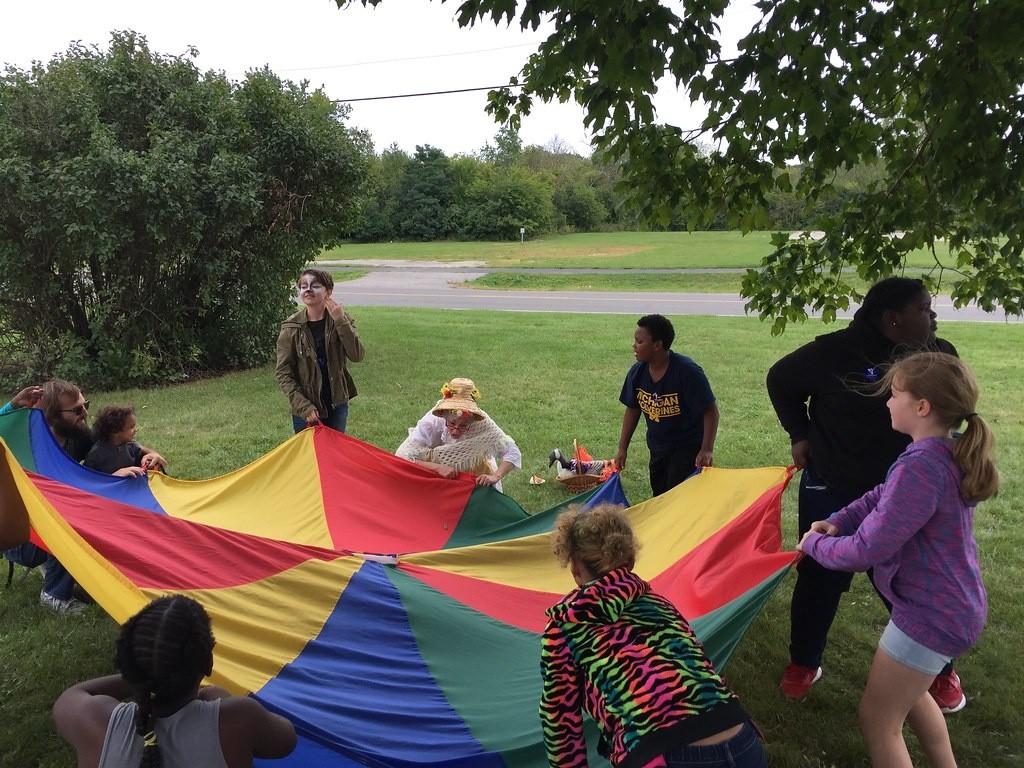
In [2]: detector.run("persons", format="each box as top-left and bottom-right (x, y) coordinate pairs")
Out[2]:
(6, 380), (95, 615)
(84, 406), (168, 477)
(275, 269), (365, 436)
(54, 594), (297, 767)
(767, 278), (966, 714)
(613, 315), (719, 498)
(0, 386), (43, 415)
(395, 378), (521, 493)
(540, 505), (767, 768)
(794, 352), (999, 768)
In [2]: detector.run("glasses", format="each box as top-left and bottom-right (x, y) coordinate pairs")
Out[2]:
(54, 400), (91, 414)
(446, 420), (470, 432)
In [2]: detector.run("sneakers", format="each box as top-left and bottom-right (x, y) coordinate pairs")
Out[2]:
(929, 670), (968, 713)
(40, 588), (86, 613)
(780, 663), (822, 699)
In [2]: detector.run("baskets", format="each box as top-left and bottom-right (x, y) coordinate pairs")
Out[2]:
(556, 439), (606, 492)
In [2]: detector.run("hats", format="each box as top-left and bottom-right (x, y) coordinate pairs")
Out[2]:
(432, 378), (485, 422)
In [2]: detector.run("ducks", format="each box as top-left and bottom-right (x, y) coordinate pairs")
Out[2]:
(548, 449), (615, 478)
